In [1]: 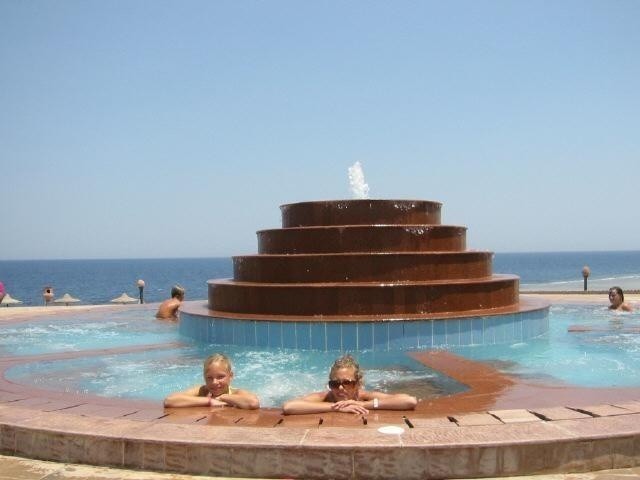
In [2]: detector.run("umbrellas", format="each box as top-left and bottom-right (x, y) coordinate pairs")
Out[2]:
(112, 293), (138, 303)
(2, 293), (24, 307)
(55, 293), (80, 306)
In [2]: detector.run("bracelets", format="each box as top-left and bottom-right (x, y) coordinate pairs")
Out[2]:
(207, 396), (212, 407)
(374, 397), (381, 410)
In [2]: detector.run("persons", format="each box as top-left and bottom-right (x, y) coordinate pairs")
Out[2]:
(157, 284), (186, 321)
(44, 289), (53, 305)
(607, 285), (633, 313)
(164, 352), (260, 410)
(283, 354), (419, 416)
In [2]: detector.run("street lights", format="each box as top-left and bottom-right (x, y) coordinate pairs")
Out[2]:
(582, 266), (590, 291)
(138, 280), (145, 304)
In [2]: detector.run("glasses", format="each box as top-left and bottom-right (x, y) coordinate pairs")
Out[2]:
(329, 379), (358, 388)
